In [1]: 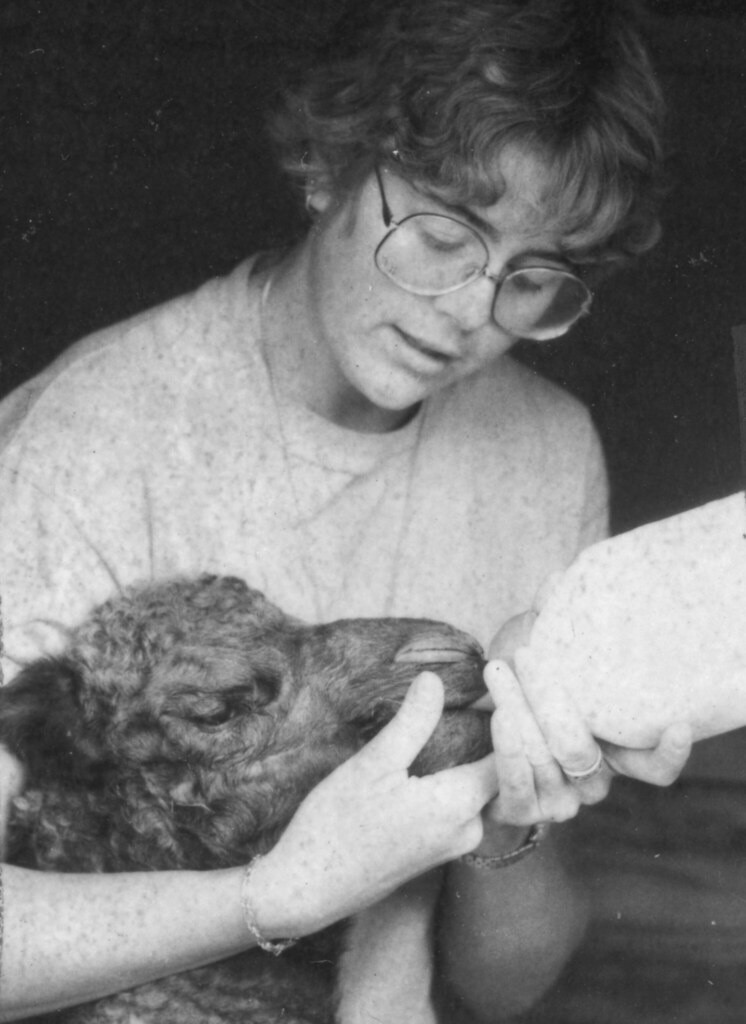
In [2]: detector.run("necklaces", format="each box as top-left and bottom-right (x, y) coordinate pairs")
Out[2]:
(256, 271), (430, 622)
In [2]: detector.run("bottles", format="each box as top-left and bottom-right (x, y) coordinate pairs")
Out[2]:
(474, 491), (746, 747)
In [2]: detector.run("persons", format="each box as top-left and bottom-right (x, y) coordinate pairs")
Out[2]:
(0, 0), (693, 1024)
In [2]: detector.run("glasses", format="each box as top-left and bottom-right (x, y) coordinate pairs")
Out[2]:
(372, 162), (594, 343)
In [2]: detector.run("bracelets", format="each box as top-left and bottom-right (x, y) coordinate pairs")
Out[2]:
(456, 822), (548, 868)
(239, 854), (299, 954)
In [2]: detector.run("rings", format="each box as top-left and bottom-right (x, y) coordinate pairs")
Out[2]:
(563, 746), (604, 785)
